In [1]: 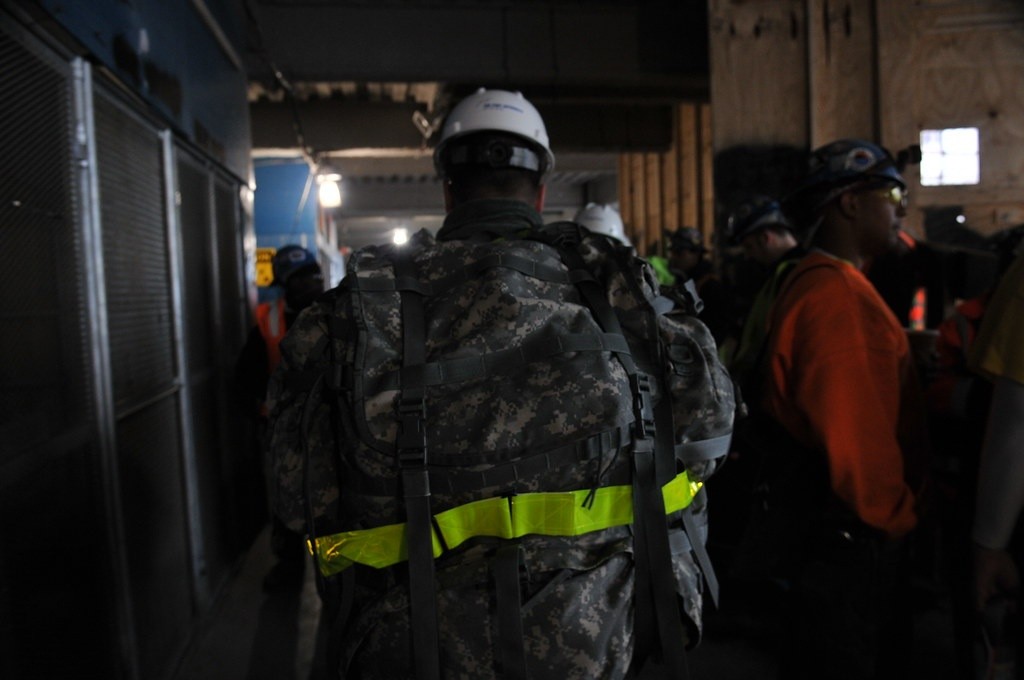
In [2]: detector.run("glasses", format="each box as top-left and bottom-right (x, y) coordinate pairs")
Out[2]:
(858, 187), (903, 203)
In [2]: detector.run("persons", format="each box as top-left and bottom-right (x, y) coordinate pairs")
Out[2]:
(264, 85), (734, 680)
(237, 244), (326, 612)
(570, 138), (1024, 680)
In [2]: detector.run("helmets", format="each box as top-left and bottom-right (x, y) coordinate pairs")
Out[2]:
(270, 245), (321, 287)
(665, 227), (708, 252)
(432, 87), (556, 186)
(733, 195), (783, 244)
(574, 202), (630, 245)
(807, 139), (900, 209)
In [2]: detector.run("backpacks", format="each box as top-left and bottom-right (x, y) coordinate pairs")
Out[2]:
(301, 228), (709, 680)
